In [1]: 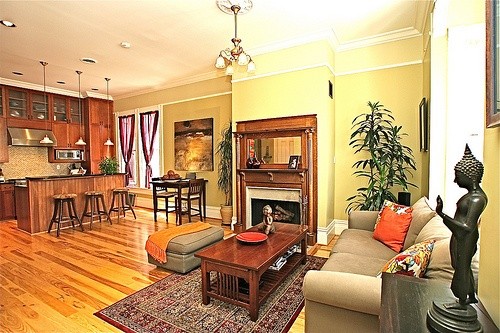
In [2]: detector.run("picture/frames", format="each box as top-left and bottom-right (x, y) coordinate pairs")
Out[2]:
(419, 97), (427, 152)
(287, 156), (299, 169)
(485, 0), (500, 128)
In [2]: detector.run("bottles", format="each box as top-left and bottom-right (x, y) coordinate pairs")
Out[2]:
(70, 105), (72, 112)
(60, 104), (64, 112)
(0, 169), (5, 183)
(54, 103), (57, 112)
(246, 152), (260, 168)
(260, 156), (265, 164)
(54, 112), (66, 120)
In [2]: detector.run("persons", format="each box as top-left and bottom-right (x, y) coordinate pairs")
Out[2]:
(261, 205), (274, 234)
(436, 144), (488, 310)
(290, 158), (296, 168)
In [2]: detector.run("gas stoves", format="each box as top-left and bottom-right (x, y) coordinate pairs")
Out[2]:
(6, 178), (27, 184)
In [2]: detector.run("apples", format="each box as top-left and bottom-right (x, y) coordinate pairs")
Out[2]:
(163, 170), (180, 179)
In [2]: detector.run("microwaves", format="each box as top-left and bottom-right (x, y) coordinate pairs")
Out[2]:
(56, 150), (80, 160)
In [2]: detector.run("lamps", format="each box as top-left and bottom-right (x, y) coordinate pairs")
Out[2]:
(39, 62), (53, 144)
(216, 0), (256, 76)
(74, 70), (86, 145)
(104, 77), (114, 146)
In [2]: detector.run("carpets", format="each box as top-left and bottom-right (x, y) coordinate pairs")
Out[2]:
(93, 250), (328, 333)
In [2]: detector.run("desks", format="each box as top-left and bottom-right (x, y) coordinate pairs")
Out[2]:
(149, 179), (208, 225)
(194, 222), (311, 322)
(378, 271), (500, 333)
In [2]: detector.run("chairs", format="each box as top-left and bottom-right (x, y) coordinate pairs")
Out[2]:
(152, 177), (205, 226)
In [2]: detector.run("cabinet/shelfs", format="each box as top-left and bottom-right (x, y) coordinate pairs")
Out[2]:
(0, 83), (116, 175)
(233, 126), (312, 174)
(0, 183), (16, 221)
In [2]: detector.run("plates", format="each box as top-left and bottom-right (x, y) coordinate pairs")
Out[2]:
(163, 179), (180, 183)
(236, 232), (268, 245)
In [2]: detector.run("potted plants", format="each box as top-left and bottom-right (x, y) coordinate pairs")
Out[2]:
(214, 121), (233, 224)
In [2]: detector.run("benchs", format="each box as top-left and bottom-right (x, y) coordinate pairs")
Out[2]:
(145, 221), (224, 275)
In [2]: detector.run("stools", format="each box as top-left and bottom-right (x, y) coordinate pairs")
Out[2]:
(48, 188), (136, 237)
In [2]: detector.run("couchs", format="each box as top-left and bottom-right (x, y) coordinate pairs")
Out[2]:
(301, 210), (479, 333)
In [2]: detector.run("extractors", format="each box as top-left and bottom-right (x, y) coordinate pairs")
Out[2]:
(8, 127), (58, 147)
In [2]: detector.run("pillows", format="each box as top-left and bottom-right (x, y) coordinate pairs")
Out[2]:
(372, 199), (436, 279)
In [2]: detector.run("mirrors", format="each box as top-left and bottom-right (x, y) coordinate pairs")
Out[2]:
(245, 136), (302, 164)
